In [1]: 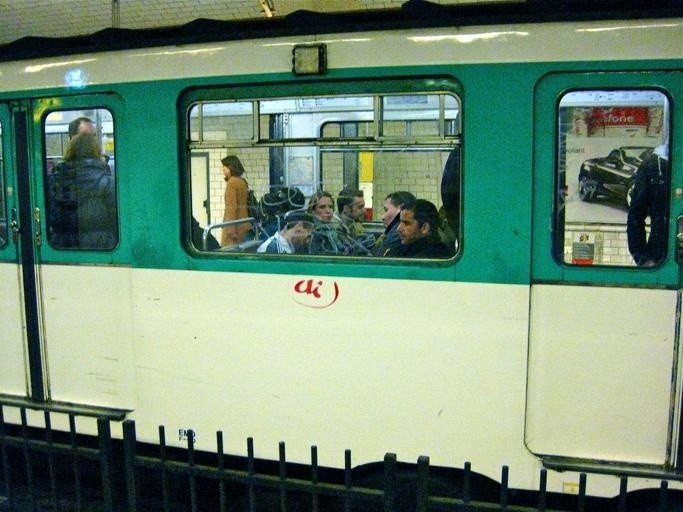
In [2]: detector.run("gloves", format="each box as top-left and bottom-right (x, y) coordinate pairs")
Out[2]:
(353, 238), (374, 253)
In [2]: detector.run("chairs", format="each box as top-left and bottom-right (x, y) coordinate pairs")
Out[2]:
(200, 206), (461, 259)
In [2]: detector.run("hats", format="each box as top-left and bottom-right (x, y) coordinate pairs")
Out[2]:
(284, 210), (317, 227)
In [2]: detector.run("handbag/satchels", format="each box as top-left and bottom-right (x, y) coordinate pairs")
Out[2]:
(247, 189), (260, 220)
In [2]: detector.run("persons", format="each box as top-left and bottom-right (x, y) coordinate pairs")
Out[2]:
(255, 184), (452, 258)
(66, 117), (100, 142)
(219, 155), (250, 247)
(627, 142), (668, 265)
(48, 132), (117, 251)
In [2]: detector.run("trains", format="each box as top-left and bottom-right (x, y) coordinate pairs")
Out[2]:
(0, 0), (682, 511)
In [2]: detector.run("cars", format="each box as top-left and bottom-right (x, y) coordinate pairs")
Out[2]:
(576, 144), (658, 208)
